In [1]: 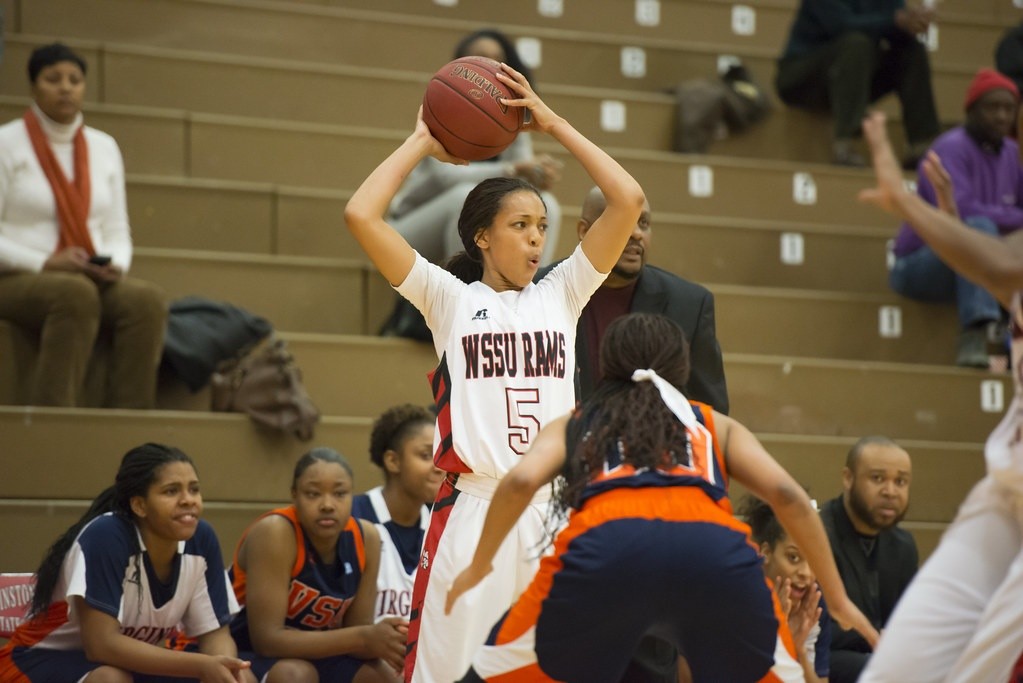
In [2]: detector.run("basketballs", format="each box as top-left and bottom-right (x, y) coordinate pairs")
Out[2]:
(422, 56), (524, 162)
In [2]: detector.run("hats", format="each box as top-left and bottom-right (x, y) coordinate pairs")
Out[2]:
(964, 67), (1019, 110)
(29, 43), (87, 83)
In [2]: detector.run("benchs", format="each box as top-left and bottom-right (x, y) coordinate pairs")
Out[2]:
(0, 0), (1023, 570)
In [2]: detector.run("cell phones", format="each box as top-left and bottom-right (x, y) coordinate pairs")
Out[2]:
(86, 257), (112, 267)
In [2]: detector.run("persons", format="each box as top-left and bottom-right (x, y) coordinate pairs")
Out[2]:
(888, 69), (1023, 369)
(351, 404), (435, 683)
(229, 449), (406, 683)
(447, 315), (878, 683)
(2, 444), (259, 683)
(343, 62), (646, 683)
(582, 0), (1023, 683)
(0, 43), (168, 408)
(377, 30), (561, 278)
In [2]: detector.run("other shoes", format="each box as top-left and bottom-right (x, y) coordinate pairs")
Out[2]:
(952, 322), (990, 368)
(901, 141), (930, 170)
(832, 144), (867, 168)
(989, 311), (1012, 353)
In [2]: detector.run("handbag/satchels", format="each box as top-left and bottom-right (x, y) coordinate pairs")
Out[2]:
(212, 338), (319, 440)
